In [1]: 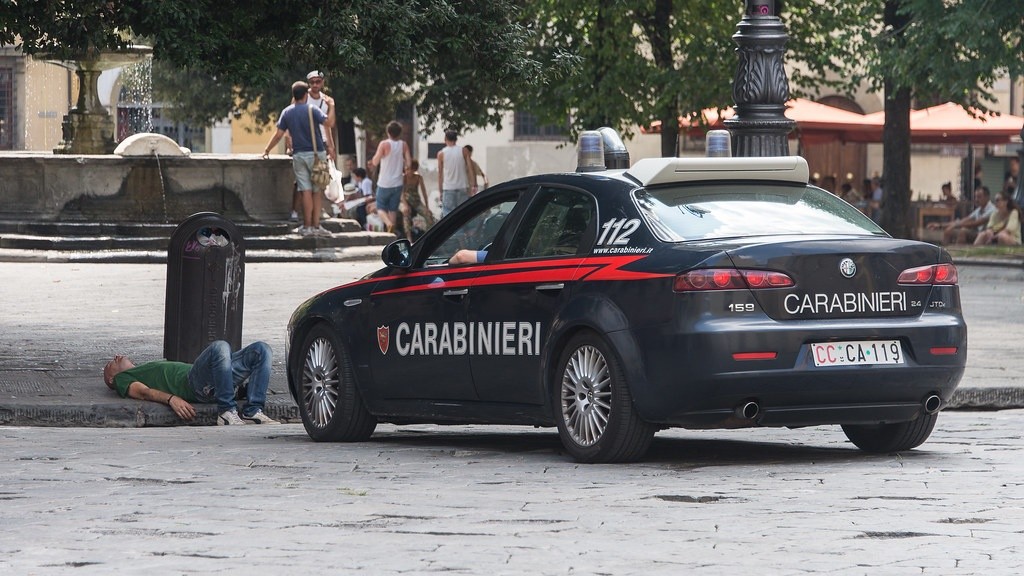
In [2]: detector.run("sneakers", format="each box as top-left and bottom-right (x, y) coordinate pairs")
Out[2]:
(217, 407), (281, 425)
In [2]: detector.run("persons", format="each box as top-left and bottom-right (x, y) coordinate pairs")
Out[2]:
(284, 70), (335, 220)
(389, 245), (407, 266)
(810, 158), (1023, 247)
(449, 249), (488, 265)
(398, 160), (434, 243)
(104, 341), (280, 425)
(437, 132), (478, 218)
(371, 122), (411, 234)
(464, 146), (488, 191)
(261, 81), (335, 235)
(340, 157), (380, 223)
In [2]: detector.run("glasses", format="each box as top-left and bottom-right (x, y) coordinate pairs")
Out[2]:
(309, 80), (321, 85)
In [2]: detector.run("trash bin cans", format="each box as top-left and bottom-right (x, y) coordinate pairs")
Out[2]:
(163, 212), (245, 363)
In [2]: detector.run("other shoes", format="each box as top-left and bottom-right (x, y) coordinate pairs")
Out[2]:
(292, 224), (331, 235)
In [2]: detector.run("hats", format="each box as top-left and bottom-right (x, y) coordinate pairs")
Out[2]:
(307, 70), (324, 79)
(343, 182), (358, 195)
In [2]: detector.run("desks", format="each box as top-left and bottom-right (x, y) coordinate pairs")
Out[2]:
(867, 201), (958, 242)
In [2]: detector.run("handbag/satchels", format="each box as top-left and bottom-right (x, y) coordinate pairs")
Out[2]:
(324, 158), (344, 204)
(311, 160), (333, 187)
(365, 212), (385, 232)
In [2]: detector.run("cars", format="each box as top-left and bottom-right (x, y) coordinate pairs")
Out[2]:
(285, 157), (965, 468)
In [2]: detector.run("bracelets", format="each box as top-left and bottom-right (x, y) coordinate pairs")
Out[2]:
(167, 395), (174, 406)
(940, 222), (942, 229)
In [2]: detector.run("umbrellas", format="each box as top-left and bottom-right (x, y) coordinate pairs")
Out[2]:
(640, 98), (1024, 213)
